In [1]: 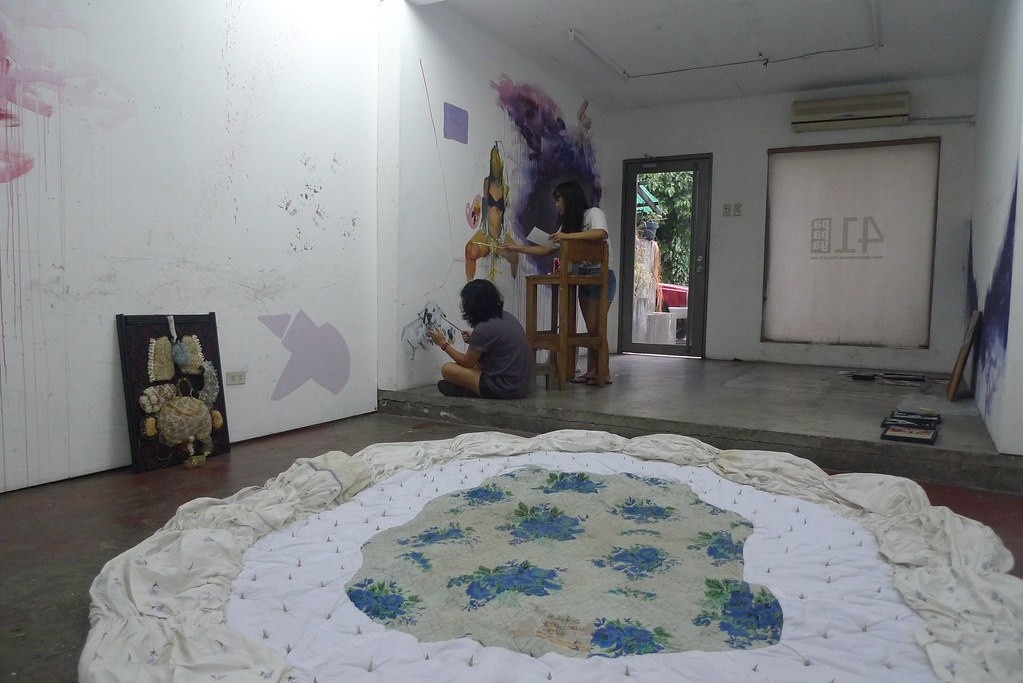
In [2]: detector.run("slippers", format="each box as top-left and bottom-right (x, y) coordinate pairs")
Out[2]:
(570, 374), (612, 385)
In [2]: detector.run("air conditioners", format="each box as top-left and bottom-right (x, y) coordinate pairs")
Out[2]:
(789, 91), (912, 132)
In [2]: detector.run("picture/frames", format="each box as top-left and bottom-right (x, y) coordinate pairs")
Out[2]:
(880, 425), (939, 446)
(881, 416), (937, 428)
(946, 308), (983, 402)
(114, 311), (234, 469)
(891, 410), (941, 425)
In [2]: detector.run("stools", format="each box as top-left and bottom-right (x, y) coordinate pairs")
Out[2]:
(525, 237), (610, 390)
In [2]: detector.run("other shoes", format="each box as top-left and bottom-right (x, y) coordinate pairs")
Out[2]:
(437, 380), (475, 398)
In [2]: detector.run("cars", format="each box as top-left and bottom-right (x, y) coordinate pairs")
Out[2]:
(653, 282), (689, 340)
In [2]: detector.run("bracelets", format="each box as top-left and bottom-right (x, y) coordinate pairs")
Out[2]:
(441, 343), (449, 351)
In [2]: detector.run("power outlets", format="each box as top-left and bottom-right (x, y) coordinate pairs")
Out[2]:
(722, 204), (731, 217)
(226, 371), (247, 384)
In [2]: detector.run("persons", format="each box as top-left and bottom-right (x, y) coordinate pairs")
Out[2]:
(498, 181), (617, 384)
(425, 279), (529, 400)
